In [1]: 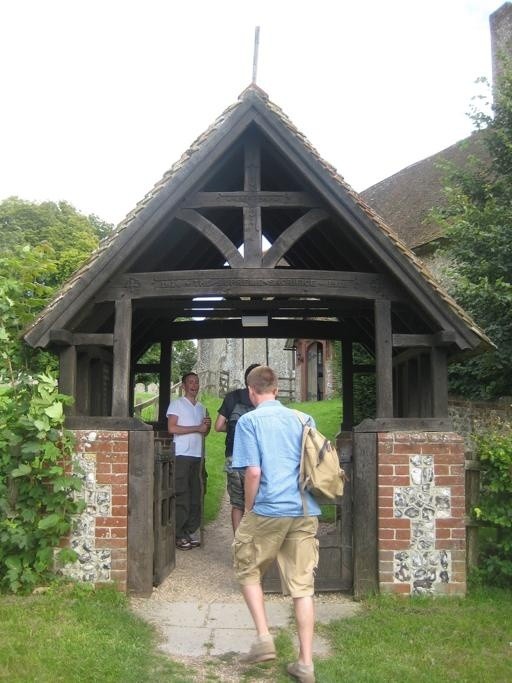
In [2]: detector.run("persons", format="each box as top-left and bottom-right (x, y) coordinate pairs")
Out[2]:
(165, 370), (210, 550)
(213, 361), (260, 539)
(229, 365), (348, 683)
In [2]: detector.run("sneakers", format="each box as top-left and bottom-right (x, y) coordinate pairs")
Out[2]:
(188, 539), (200, 547)
(174, 538), (191, 550)
(286, 661), (316, 683)
(238, 637), (278, 666)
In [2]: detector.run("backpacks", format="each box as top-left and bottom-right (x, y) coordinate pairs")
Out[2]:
(300, 424), (344, 501)
(227, 402), (252, 445)
